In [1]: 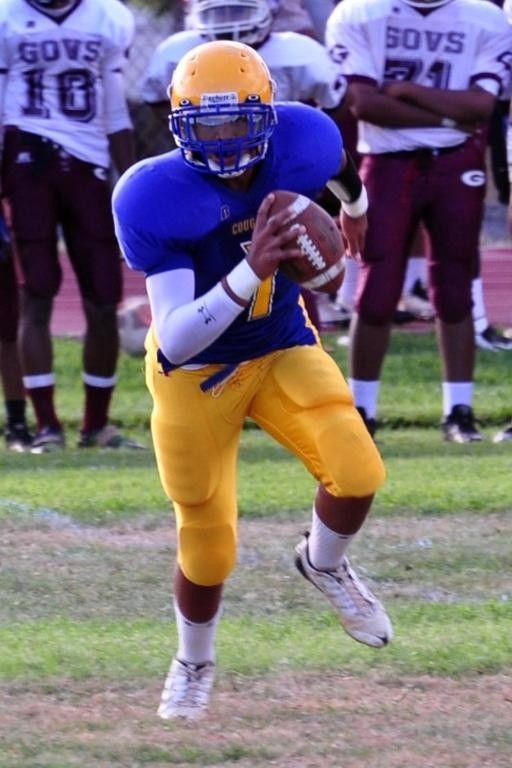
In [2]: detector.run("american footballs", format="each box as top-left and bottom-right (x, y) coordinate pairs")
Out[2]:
(265, 191), (345, 294)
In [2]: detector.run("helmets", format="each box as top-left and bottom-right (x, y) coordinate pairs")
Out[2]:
(193, 0), (272, 45)
(168, 41), (278, 179)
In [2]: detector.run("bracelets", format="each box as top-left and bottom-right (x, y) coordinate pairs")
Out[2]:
(442, 117), (455, 128)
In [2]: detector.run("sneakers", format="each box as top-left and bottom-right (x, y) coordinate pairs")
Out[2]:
(443, 405), (482, 443)
(74, 425), (142, 450)
(159, 657), (218, 720)
(5, 422), (30, 454)
(31, 425), (65, 453)
(293, 531), (394, 648)
(475, 324), (511, 350)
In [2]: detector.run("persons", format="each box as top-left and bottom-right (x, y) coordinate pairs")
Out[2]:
(326, 2), (510, 443)
(1, 2), (138, 452)
(126, 0), (364, 334)
(111, 40), (392, 721)
